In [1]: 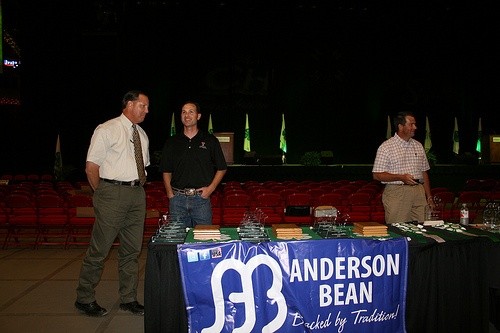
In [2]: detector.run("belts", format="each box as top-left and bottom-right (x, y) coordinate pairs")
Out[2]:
(172, 188), (203, 196)
(100, 177), (139, 186)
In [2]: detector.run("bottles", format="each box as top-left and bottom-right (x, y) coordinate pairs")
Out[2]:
(459, 203), (469, 226)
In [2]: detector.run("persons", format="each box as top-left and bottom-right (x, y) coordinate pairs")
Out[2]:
(371, 112), (436, 225)
(162, 101), (229, 226)
(74, 91), (153, 318)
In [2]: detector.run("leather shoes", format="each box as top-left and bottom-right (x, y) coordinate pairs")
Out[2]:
(75, 301), (107, 316)
(121, 301), (145, 315)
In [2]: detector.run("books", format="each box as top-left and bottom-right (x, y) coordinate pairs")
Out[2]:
(193, 224), (222, 240)
(272, 223), (302, 239)
(353, 222), (388, 236)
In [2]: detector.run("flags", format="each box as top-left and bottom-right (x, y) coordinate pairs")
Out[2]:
(243, 114), (251, 152)
(424, 116), (432, 154)
(386, 115), (391, 139)
(452, 117), (459, 152)
(170, 113), (176, 136)
(280, 114), (287, 153)
(208, 114), (213, 134)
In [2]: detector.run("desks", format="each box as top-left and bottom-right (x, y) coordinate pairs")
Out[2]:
(145, 228), (500, 333)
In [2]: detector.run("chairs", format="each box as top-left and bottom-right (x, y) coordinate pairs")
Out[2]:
(0, 174), (500, 248)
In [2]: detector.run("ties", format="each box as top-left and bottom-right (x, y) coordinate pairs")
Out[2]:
(131, 125), (147, 186)
